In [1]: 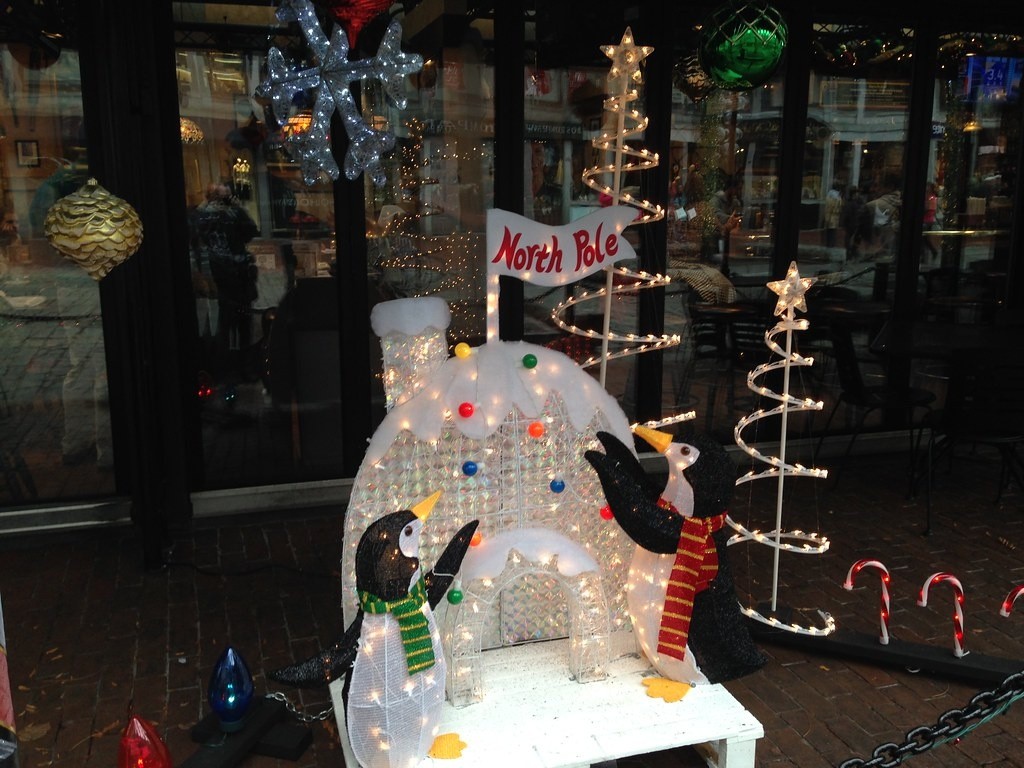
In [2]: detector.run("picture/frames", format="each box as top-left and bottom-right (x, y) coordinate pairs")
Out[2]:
(15, 140), (40, 168)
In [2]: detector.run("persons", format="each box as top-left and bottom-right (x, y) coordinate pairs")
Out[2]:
(700, 179), (741, 266)
(31, 148), (939, 374)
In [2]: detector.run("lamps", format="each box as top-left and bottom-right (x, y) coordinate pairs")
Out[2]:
(278, 109), (331, 143)
(963, 113), (983, 132)
(180, 117), (203, 145)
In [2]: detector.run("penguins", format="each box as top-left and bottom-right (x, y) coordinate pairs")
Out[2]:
(583, 425), (772, 685)
(264, 490), (480, 766)
